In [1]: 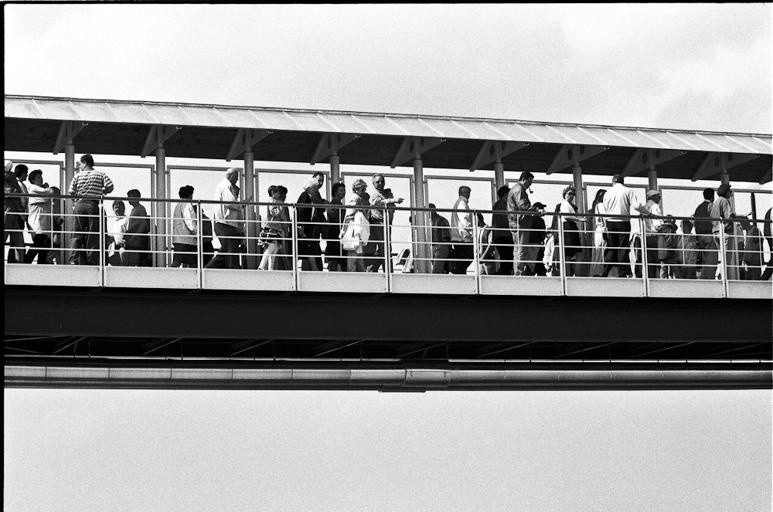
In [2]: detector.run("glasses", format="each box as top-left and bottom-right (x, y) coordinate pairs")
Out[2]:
(566, 191), (577, 197)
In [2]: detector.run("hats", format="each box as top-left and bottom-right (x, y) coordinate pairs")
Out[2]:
(646, 190), (660, 200)
